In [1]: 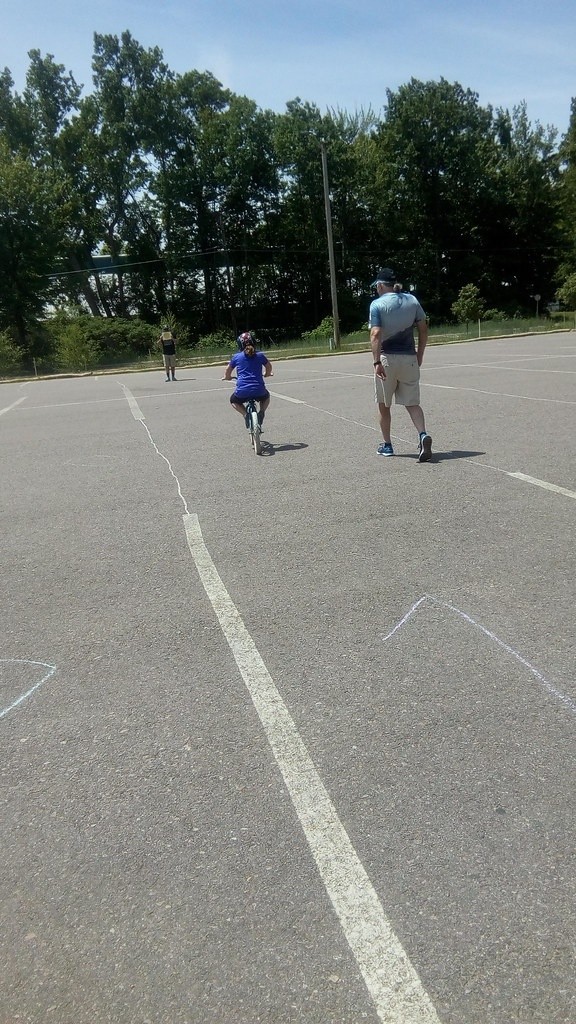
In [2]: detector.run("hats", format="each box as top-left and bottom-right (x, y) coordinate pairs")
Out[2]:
(377, 267), (396, 281)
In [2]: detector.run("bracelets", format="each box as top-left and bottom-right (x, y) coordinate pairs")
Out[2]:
(374, 361), (381, 366)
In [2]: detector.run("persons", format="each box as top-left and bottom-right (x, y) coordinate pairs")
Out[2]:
(368, 268), (432, 462)
(159, 327), (178, 382)
(224, 331), (272, 429)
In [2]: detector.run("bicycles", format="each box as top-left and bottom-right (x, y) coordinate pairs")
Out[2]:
(222, 373), (274, 455)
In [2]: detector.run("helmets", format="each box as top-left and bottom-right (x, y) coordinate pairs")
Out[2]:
(237, 333), (255, 350)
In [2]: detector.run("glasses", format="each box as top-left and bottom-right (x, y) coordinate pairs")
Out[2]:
(374, 282), (389, 289)
(163, 328), (168, 330)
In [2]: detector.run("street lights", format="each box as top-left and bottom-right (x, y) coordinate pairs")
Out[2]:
(299, 131), (340, 350)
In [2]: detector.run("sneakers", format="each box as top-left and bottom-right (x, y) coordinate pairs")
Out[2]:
(417, 434), (432, 461)
(376, 443), (394, 456)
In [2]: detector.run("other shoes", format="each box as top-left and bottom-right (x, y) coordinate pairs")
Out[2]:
(258, 411), (264, 426)
(244, 413), (251, 429)
(172, 377), (176, 381)
(164, 378), (170, 382)
